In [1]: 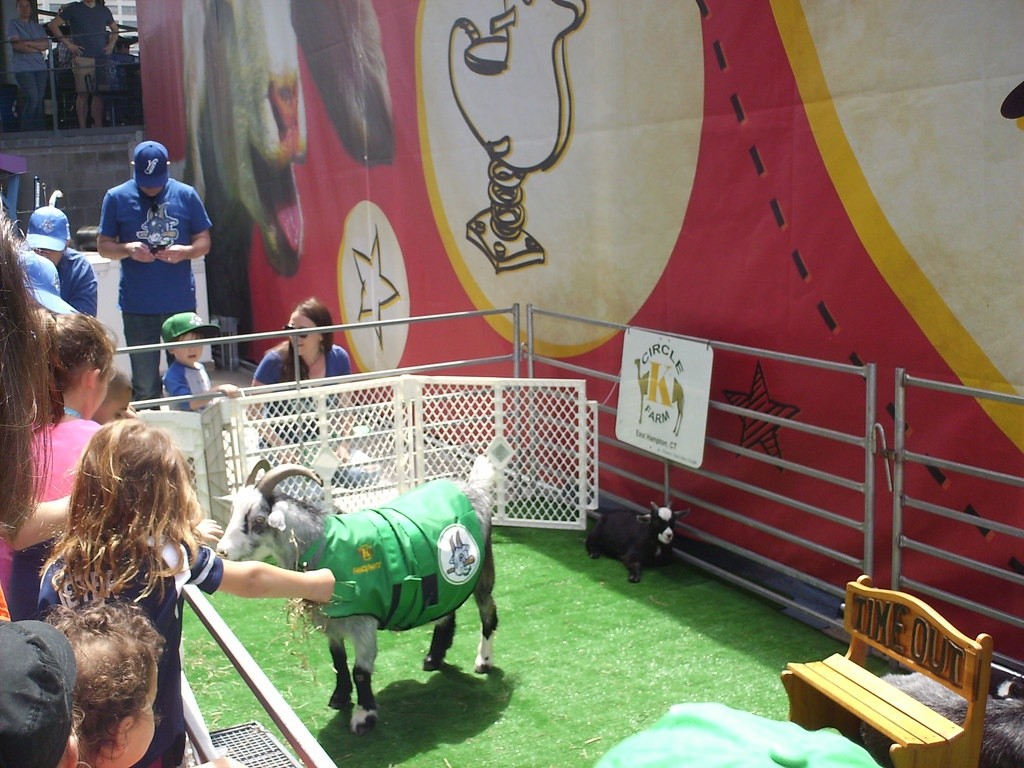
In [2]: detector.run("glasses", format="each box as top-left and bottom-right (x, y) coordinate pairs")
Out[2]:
(283, 325), (309, 339)
(151, 196), (159, 213)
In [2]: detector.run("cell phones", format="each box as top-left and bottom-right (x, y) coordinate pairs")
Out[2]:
(151, 246), (158, 255)
(157, 244), (165, 251)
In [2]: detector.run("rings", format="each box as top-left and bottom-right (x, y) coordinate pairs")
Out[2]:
(168, 257), (171, 261)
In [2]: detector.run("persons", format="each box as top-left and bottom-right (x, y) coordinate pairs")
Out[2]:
(0, 208), (336, 768)
(96, 140), (213, 412)
(244, 298), (380, 499)
(0, 0), (130, 131)
(162, 313), (240, 412)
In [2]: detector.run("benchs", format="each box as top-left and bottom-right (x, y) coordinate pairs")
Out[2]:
(780, 570), (994, 768)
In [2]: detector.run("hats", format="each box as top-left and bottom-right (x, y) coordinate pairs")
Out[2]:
(0, 620), (76, 768)
(162, 312), (221, 343)
(133, 141), (169, 188)
(17, 251), (80, 315)
(26, 206), (70, 251)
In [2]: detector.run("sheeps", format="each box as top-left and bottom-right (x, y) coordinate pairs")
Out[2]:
(209, 452), (507, 734)
(174, 0), (396, 361)
(584, 500), (691, 584)
(856, 674), (1024, 768)
